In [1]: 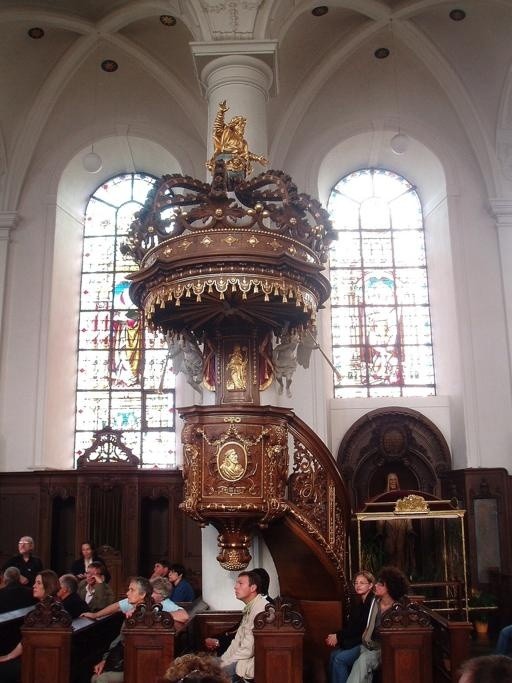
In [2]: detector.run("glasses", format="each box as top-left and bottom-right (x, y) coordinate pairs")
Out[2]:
(352, 582), (370, 586)
(84, 572), (100, 575)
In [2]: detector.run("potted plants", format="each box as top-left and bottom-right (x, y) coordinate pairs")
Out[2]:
(468, 593), (496, 633)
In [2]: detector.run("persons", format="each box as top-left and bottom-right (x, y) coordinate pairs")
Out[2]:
(457, 653), (511, 683)
(225, 345), (248, 390)
(204, 97), (273, 178)
(270, 330), (299, 400)
(163, 331), (206, 404)
(387, 472), (399, 493)
(220, 447), (244, 480)
(324, 570), (377, 683)
(1, 534), (281, 681)
(346, 565), (409, 683)
